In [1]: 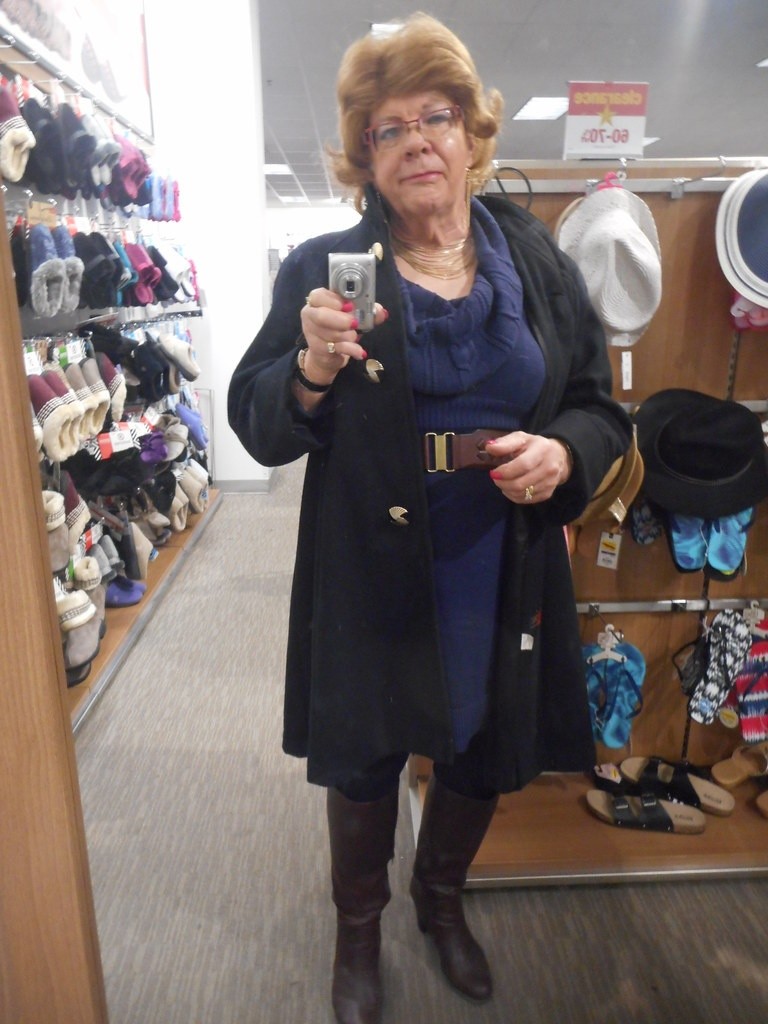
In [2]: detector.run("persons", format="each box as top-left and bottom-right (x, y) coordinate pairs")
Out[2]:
(226, 10), (633, 1024)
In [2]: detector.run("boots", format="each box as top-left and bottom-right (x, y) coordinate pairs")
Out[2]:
(409, 764), (503, 999)
(326, 782), (398, 1024)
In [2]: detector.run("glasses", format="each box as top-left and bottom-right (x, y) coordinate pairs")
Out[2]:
(359, 106), (464, 152)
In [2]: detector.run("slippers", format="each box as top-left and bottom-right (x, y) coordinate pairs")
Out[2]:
(704, 504), (754, 579)
(620, 754), (734, 816)
(0, 73), (211, 688)
(687, 604), (768, 742)
(754, 790), (768, 817)
(664, 501), (710, 572)
(711, 738), (768, 790)
(578, 641), (647, 750)
(585, 789), (706, 834)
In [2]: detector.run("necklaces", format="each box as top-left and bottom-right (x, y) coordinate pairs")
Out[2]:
(389, 227), (476, 281)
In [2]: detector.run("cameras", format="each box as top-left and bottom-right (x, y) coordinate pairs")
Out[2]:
(327, 252), (376, 333)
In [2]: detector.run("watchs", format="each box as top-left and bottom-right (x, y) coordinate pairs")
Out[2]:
(295, 348), (336, 393)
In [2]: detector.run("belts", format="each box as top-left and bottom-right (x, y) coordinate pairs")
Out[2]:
(424, 425), (522, 473)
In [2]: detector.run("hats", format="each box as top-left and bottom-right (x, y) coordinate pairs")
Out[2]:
(715, 168), (768, 310)
(632, 387), (768, 519)
(558, 187), (662, 347)
(569, 433), (644, 526)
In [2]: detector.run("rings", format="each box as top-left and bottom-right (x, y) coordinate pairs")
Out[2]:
(524, 486), (534, 501)
(328, 342), (335, 355)
(305, 296), (312, 307)
